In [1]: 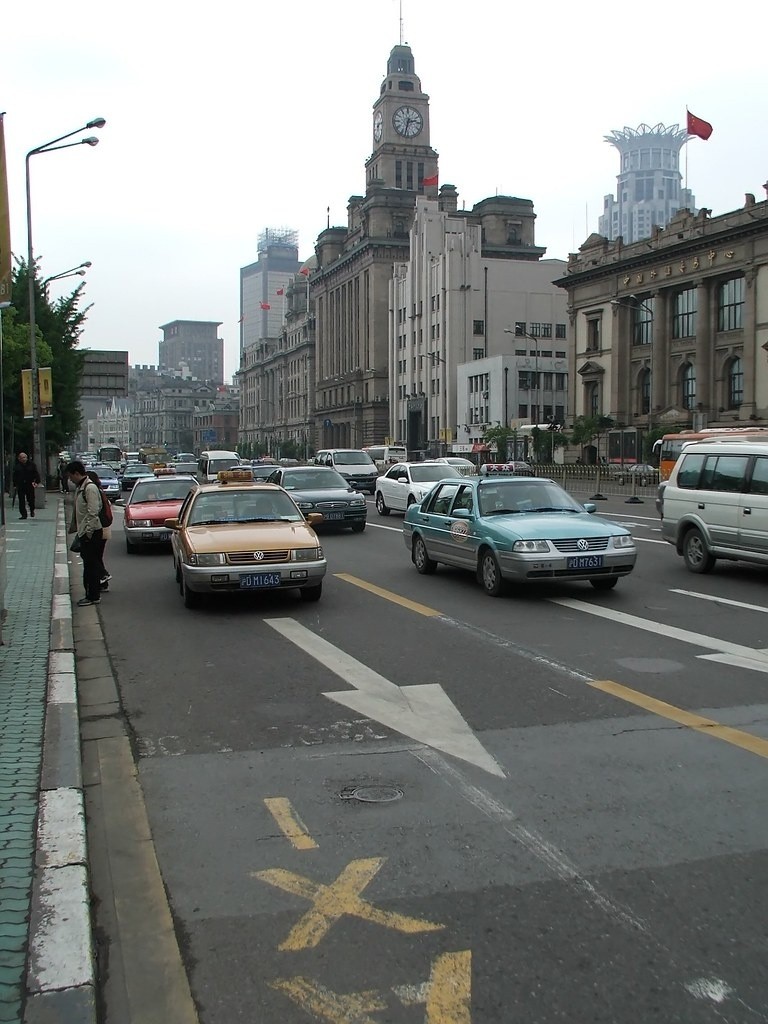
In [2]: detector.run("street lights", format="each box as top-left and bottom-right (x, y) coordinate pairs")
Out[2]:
(610, 295), (653, 428)
(366, 367), (389, 445)
(503, 324), (540, 460)
(24, 116), (108, 507)
(419, 351), (448, 456)
(335, 376), (356, 449)
(288, 391), (307, 460)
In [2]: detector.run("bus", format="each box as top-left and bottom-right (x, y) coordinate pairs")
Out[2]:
(656, 435), (768, 575)
(362, 444), (408, 464)
(651, 427), (768, 483)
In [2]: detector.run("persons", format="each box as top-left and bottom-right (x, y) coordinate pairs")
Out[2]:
(13, 452), (41, 519)
(474, 455), (605, 474)
(59, 459), (114, 606)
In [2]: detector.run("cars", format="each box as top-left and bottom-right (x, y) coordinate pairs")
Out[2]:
(115, 468), (201, 555)
(614, 463), (659, 486)
(164, 470), (325, 606)
(505, 461), (535, 476)
(374, 462), (465, 516)
(85, 467), (121, 502)
(265, 466), (368, 533)
(58, 444), (380, 497)
(435, 457), (477, 477)
(403, 464), (638, 593)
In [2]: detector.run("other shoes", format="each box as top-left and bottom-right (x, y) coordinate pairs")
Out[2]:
(19, 516), (26, 520)
(100, 572), (112, 583)
(77, 594), (102, 605)
(101, 582), (109, 592)
(30, 512), (35, 517)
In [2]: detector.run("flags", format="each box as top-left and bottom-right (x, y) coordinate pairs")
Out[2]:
(237, 316), (243, 323)
(261, 302), (271, 309)
(687, 111), (713, 140)
(276, 289), (284, 295)
(423, 175), (438, 186)
(299, 267), (309, 275)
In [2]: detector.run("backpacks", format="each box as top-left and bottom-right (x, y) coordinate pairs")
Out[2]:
(84, 482), (113, 527)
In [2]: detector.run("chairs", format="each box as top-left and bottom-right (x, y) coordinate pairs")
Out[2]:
(243, 506), (256, 514)
(203, 506), (222, 514)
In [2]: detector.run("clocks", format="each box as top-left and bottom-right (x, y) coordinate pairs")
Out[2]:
(373, 110), (382, 141)
(392, 106), (424, 138)
